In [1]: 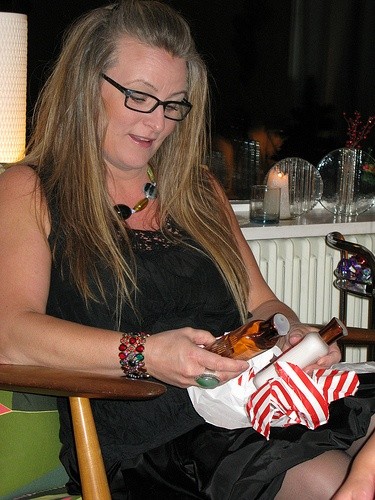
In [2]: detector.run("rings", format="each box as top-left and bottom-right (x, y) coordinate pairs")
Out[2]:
(194, 368), (220, 389)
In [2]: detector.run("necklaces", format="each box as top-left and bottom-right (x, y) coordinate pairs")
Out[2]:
(112, 160), (157, 223)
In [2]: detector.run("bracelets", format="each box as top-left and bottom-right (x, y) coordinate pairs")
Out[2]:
(118, 330), (149, 380)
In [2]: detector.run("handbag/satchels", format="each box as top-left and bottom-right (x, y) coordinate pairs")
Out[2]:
(189, 335), (360, 439)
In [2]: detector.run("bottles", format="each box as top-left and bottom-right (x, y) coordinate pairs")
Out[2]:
(252, 316), (348, 390)
(201, 313), (290, 361)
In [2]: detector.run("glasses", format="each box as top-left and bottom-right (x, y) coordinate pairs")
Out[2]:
(99, 72), (192, 121)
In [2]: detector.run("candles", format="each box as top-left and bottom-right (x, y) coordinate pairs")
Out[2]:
(266, 168), (292, 220)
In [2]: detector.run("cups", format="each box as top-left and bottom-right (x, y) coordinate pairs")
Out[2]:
(249, 185), (282, 226)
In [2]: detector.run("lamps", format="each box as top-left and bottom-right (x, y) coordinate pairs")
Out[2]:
(0, 11), (30, 166)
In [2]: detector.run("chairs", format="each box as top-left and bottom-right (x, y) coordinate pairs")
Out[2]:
(325, 230), (375, 328)
(0, 323), (375, 500)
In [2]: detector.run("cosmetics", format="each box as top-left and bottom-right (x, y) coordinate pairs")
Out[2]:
(202, 311), (288, 362)
(252, 314), (350, 389)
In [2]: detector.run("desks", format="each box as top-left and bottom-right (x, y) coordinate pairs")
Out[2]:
(230, 208), (375, 364)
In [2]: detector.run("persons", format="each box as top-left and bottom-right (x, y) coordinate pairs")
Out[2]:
(1, 0), (374, 499)
